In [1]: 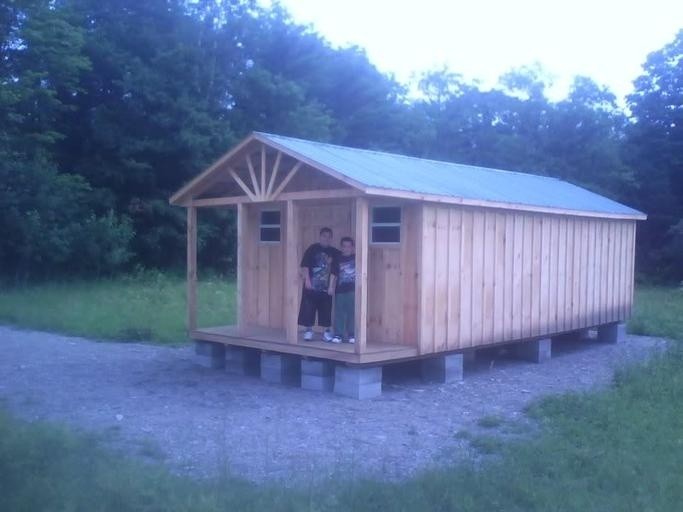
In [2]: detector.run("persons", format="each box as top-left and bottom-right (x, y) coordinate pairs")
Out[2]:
(297, 227), (338, 342)
(326, 237), (355, 344)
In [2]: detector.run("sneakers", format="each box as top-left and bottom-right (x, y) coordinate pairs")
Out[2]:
(304, 330), (355, 344)
